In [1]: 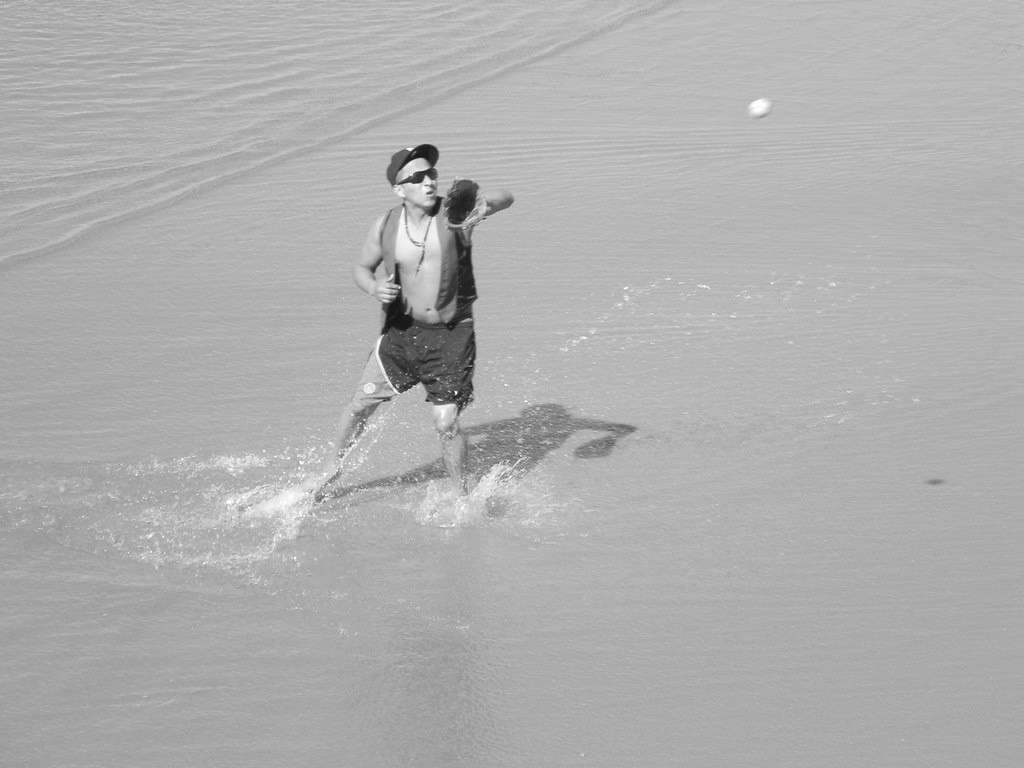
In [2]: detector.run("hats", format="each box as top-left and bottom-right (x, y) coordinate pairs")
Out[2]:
(387, 144), (439, 185)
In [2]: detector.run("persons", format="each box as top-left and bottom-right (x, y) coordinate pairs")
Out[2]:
(308, 144), (516, 506)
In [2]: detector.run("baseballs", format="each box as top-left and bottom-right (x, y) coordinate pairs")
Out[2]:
(747, 95), (777, 121)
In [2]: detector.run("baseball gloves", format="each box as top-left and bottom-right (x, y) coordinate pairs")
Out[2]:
(440, 173), (491, 235)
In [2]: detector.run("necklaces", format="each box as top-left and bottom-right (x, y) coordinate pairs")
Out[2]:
(405, 204), (431, 272)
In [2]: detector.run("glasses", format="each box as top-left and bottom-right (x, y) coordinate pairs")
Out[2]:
(397, 168), (438, 186)
